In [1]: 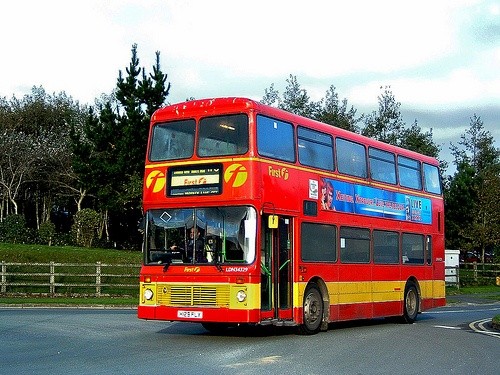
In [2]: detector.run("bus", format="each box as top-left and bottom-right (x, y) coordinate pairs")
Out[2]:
(137, 96), (447, 334)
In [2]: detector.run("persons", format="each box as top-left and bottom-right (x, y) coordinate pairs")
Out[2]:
(171, 227), (207, 263)
